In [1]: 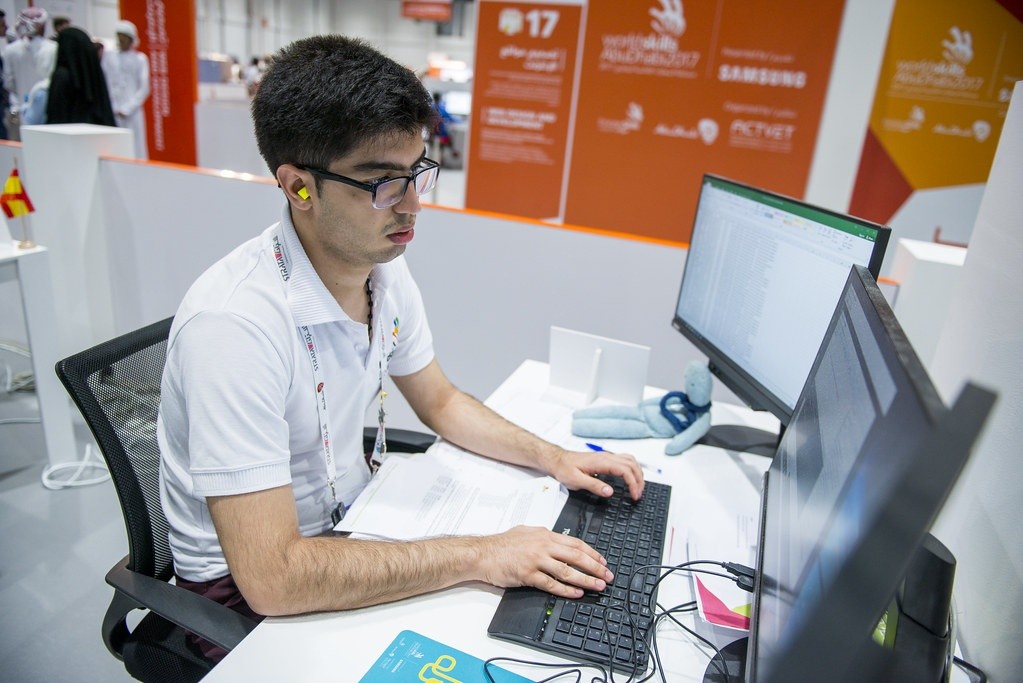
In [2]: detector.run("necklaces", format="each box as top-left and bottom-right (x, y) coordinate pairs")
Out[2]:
(365, 278), (374, 339)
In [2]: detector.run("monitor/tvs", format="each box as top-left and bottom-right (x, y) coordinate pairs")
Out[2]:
(672, 175), (1002, 683)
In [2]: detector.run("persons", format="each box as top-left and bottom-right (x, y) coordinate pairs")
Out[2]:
(154, 33), (645, 665)
(424, 92), (461, 165)
(0, 0), (154, 162)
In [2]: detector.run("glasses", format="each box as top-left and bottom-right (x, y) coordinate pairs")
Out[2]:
(277, 157), (442, 210)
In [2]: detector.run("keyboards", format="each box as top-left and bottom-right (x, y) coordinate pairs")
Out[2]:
(486, 474), (673, 676)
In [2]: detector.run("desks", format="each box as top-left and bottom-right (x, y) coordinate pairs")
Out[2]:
(198, 360), (781, 683)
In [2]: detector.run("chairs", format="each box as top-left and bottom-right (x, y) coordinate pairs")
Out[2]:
(55, 315), (436, 683)
